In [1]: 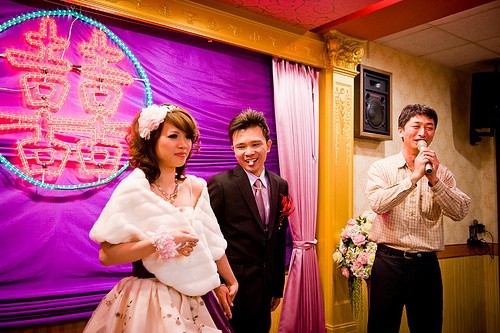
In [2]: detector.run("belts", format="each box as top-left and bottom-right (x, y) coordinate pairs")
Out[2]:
(379, 242), (425, 260)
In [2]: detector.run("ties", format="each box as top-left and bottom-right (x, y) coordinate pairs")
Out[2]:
(253, 180), (269, 226)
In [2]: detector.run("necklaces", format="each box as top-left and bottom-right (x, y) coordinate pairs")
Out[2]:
(154, 179), (179, 203)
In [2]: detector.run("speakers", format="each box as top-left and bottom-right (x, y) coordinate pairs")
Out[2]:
(471, 71), (500, 129)
(362, 71), (391, 135)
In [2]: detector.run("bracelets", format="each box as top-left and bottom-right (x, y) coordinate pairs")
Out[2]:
(153, 233), (177, 262)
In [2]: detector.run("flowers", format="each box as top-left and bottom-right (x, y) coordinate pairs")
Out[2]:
(278, 194), (295, 229)
(332, 211), (377, 317)
(138, 104), (168, 140)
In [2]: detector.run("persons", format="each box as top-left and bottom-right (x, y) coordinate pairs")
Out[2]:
(82, 104), (239, 333)
(206, 108), (288, 333)
(366, 104), (471, 333)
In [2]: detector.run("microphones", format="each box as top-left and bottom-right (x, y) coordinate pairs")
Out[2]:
(417, 140), (433, 174)
